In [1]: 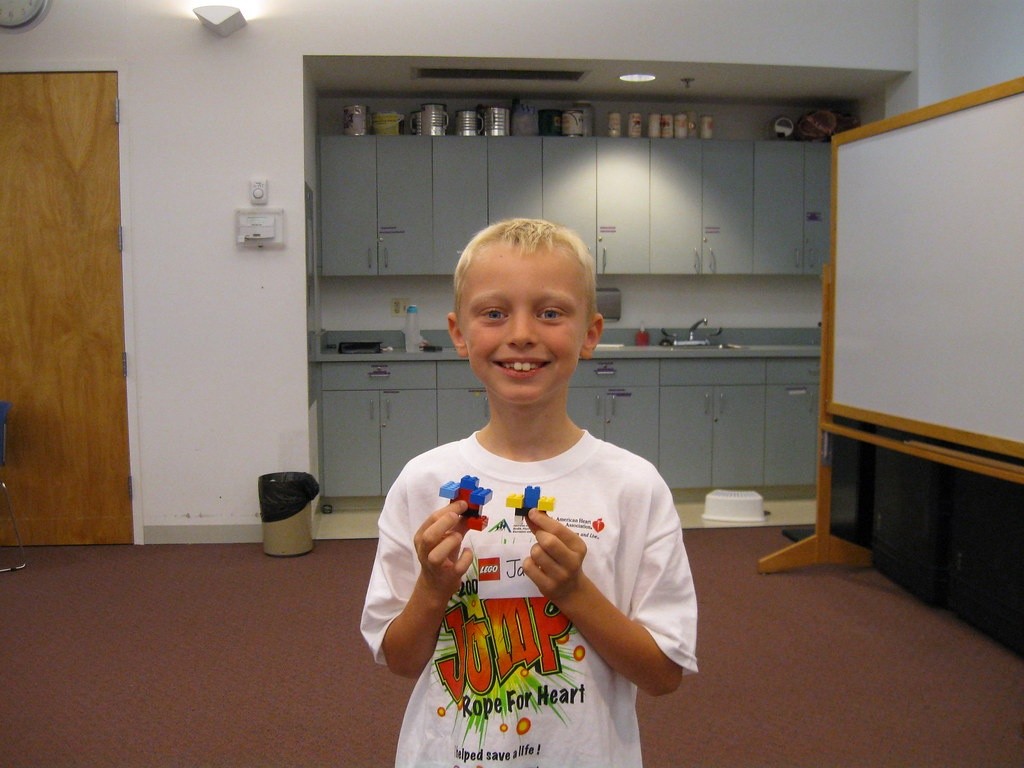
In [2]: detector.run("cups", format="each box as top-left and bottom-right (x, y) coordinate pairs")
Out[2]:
(538, 109), (563, 135)
(410, 103), (449, 137)
(484, 108), (510, 136)
(454, 111), (484, 137)
(561, 110), (583, 137)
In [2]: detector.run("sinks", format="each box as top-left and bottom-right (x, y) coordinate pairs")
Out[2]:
(662, 343), (742, 349)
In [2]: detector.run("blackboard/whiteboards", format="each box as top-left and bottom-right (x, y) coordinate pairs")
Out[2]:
(823, 75), (1023, 461)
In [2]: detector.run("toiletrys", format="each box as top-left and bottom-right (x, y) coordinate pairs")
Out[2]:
(635, 320), (649, 346)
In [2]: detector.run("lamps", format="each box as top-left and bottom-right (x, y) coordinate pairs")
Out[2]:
(192, 6), (247, 35)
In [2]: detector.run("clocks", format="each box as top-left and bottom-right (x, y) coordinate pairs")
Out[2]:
(0, 0), (52, 33)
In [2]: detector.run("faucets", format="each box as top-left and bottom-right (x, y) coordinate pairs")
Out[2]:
(659, 317), (723, 346)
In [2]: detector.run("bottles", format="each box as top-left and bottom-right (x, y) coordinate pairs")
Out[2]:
(476, 95), (537, 135)
(674, 111), (687, 139)
(662, 110), (674, 138)
(628, 111), (642, 137)
(404, 304), (421, 353)
(573, 102), (594, 137)
(700, 114), (713, 140)
(608, 111), (621, 137)
(687, 110), (699, 138)
(648, 111), (662, 138)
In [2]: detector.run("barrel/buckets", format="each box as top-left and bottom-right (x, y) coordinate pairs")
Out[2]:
(342, 105), (368, 136)
(372, 112), (404, 136)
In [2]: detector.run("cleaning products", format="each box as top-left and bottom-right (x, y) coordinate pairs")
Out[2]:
(405, 304), (420, 353)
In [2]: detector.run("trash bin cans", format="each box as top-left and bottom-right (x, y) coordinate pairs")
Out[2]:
(258, 472), (314, 557)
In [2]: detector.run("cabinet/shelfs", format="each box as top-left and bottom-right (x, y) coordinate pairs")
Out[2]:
(769, 361), (827, 484)
(664, 357), (769, 488)
(438, 362), (503, 451)
(324, 136), (433, 278)
(564, 357), (660, 468)
(645, 140), (755, 275)
(548, 137), (648, 274)
(752, 141), (834, 274)
(431, 136), (547, 279)
(324, 362), (437, 495)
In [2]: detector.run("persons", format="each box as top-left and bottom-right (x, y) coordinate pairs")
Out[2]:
(360, 220), (699, 768)
